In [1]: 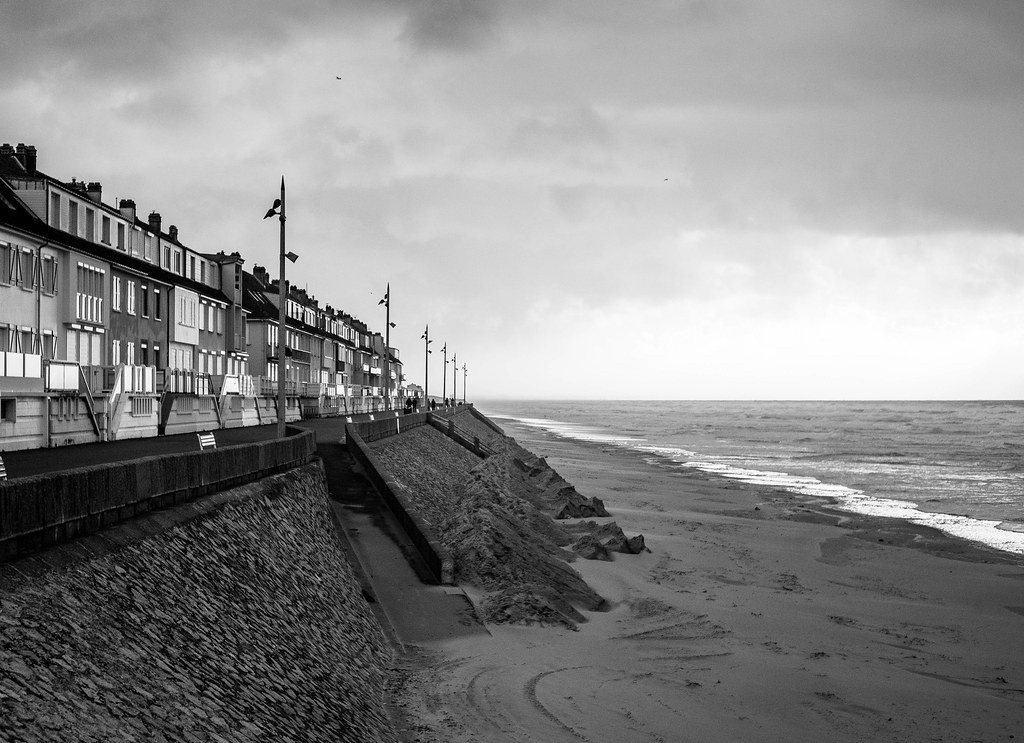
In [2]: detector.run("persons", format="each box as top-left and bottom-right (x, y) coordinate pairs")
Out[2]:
(406, 398), (417, 412)
(428, 399), (436, 411)
(445, 398), (454, 408)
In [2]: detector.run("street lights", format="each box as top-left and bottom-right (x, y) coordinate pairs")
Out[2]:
(461, 362), (467, 405)
(378, 282), (396, 412)
(451, 352), (458, 407)
(440, 342), (449, 409)
(262, 176), (300, 442)
(419, 324), (433, 411)
